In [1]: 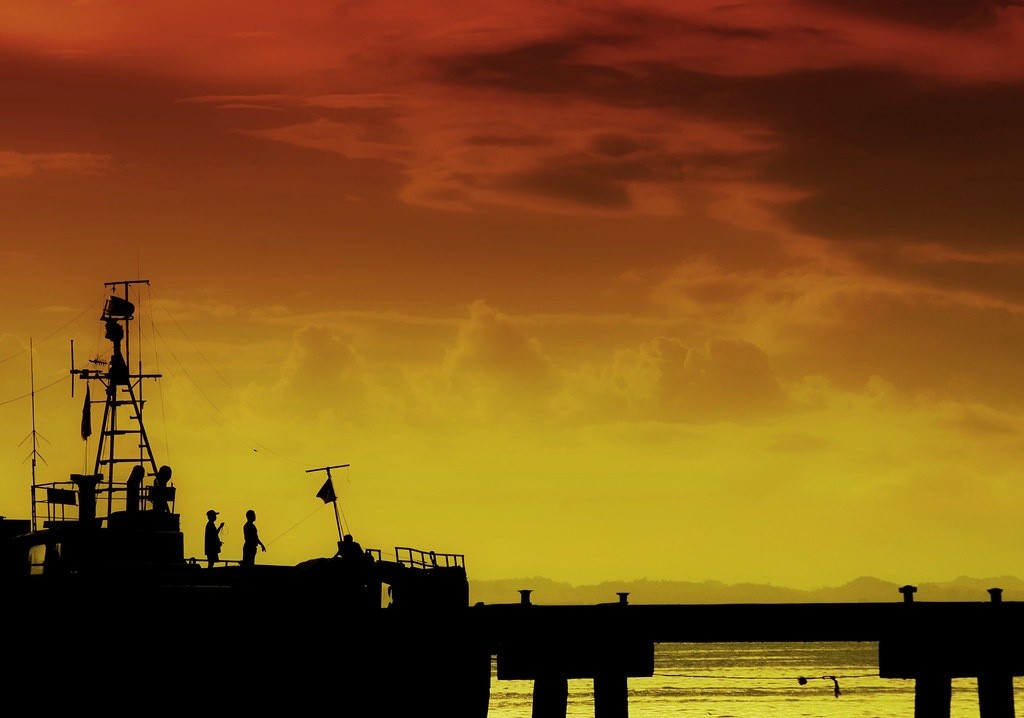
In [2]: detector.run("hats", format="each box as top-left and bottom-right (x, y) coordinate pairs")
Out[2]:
(206, 510), (219, 516)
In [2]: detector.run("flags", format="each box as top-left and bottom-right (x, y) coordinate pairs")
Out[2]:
(82, 385), (92, 440)
(316, 479), (337, 504)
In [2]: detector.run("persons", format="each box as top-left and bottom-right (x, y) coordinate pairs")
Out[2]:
(333, 534), (365, 560)
(243, 510), (266, 565)
(205, 510), (225, 568)
